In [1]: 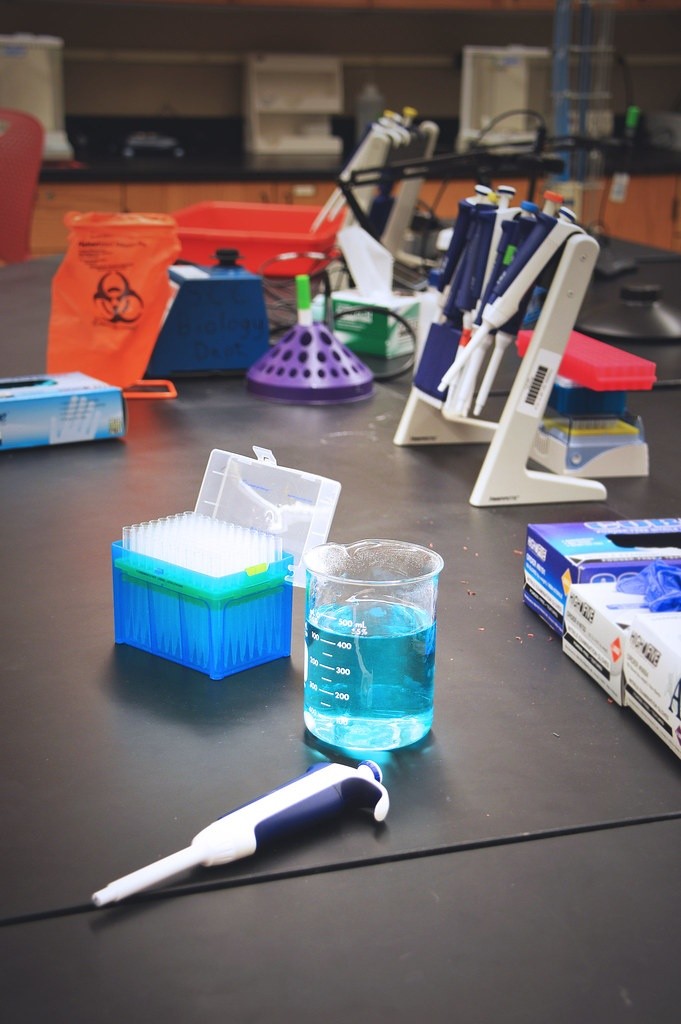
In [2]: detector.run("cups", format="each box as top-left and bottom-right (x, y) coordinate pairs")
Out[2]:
(302, 539), (445, 749)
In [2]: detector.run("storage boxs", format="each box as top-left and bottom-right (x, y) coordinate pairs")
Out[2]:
(167, 194), (347, 281)
(520, 516), (681, 760)
(109, 443), (341, 682)
(0, 370), (129, 453)
